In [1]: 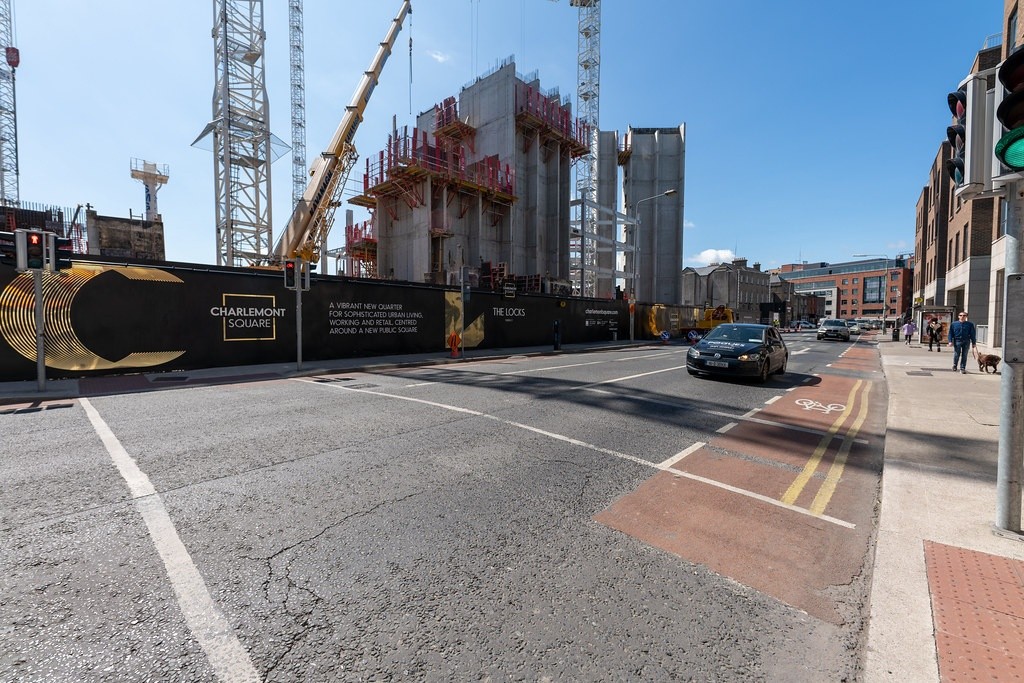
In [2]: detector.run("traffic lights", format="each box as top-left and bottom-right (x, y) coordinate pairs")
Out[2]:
(946, 72), (988, 197)
(284, 259), (296, 289)
(305, 262), (318, 290)
(51, 234), (73, 273)
(24, 228), (46, 273)
(992, 43), (1024, 179)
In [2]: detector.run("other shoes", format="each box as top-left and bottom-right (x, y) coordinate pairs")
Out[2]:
(905, 341), (907, 345)
(908, 343), (910, 345)
(937, 347), (940, 352)
(952, 365), (957, 371)
(928, 349), (932, 351)
(960, 368), (966, 374)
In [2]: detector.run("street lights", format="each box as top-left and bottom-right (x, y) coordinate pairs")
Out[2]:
(853, 254), (887, 335)
(629, 189), (678, 343)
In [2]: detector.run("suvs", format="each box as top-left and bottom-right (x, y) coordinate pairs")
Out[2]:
(817, 318), (828, 328)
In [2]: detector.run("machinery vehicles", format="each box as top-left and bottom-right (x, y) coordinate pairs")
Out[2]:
(249, 0), (414, 274)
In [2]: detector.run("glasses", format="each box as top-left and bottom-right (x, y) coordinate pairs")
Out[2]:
(959, 315), (966, 316)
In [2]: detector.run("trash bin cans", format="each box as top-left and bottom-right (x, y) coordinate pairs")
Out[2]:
(892, 329), (900, 342)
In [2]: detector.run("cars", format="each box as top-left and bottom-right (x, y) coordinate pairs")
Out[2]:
(686, 323), (788, 384)
(848, 317), (883, 335)
(790, 320), (799, 328)
(816, 317), (852, 342)
(799, 321), (816, 329)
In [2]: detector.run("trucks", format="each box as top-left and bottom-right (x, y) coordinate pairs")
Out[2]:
(682, 303), (740, 344)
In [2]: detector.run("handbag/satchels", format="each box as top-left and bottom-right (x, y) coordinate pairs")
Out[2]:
(937, 334), (942, 340)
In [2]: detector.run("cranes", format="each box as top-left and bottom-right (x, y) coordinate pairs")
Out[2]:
(569, 0), (601, 297)
(288, 0), (307, 213)
(211, 0), (272, 269)
(0, 0), (21, 230)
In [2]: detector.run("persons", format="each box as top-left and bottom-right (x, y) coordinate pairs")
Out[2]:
(774, 319), (780, 328)
(926, 318), (943, 352)
(901, 320), (913, 345)
(948, 312), (977, 374)
